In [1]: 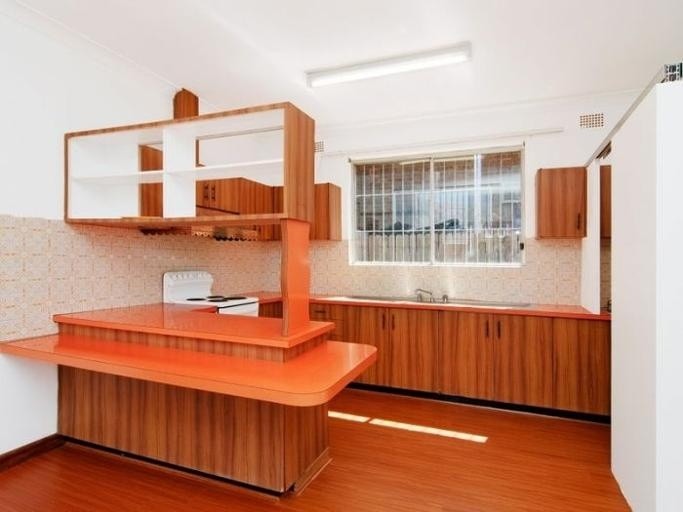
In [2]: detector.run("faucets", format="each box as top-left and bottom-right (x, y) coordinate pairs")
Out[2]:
(414, 288), (434, 302)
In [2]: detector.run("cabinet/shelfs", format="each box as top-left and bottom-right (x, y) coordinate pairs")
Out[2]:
(537, 167), (588, 239)
(308, 302), (343, 342)
(438, 309), (552, 409)
(64, 102), (315, 227)
(140, 145), (342, 241)
(260, 301), (283, 318)
(552, 317), (611, 417)
(343, 305), (438, 395)
(600, 165), (611, 238)
